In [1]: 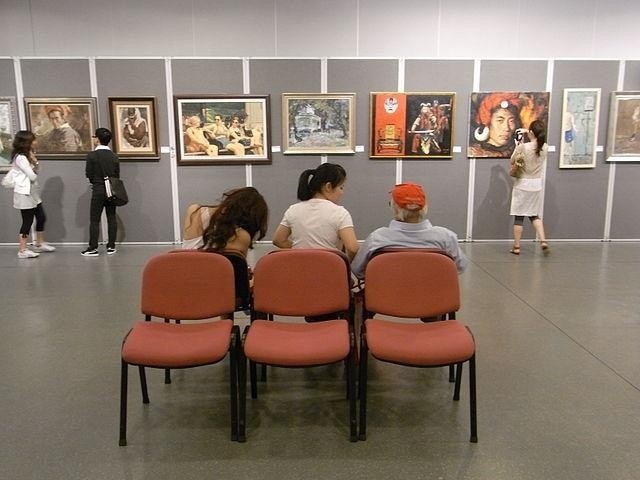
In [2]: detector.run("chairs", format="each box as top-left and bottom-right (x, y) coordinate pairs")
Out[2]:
(119, 251), (238, 446)
(358, 252), (478, 443)
(362, 246), (457, 382)
(237, 249), (358, 443)
(252, 249), (356, 381)
(165, 248), (257, 384)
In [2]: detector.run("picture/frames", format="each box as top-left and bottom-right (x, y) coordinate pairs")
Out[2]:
(467, 91), (551, 158)
(108, 96), (161, 162)
(23, 97), (100, 161)
(174, 94), (273, 165)
(0, 96), (21, 174)
(558, 88), (601, 168)
(368, 92), (456, 159)
(604, 91), (640, 161)
(281, 92), (356, 154)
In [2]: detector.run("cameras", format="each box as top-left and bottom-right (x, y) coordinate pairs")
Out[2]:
(516, 131), (523, 142)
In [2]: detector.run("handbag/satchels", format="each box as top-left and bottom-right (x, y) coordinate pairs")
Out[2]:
(508, 156), (526, 179)
(102, 175), (129, 207)
(2, 169), (15, 189)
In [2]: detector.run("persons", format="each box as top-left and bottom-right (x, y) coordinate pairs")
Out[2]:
(183, 110), (263, 157)
(411, 104), (441, 155)
(123, 107), (151, 148)
(509, 120), (551, 256)
(42, 105), (83, 151)
(272, 164), (361, 323)
(184, 186), (268, 318)
(8, 130), (56, 259)
(79, 128), (120, 257)
(349, 181), (470, 322)
(469, 92), (524, 157)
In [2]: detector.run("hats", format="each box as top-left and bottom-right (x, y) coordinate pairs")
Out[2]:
(388, 181), (426, 212)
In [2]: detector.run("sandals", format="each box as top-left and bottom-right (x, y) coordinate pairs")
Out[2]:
(540, 240), (551, 257)
(508, 245), (521, 255)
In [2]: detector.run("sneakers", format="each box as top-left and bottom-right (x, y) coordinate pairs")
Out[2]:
(16, 248), (40, 259)
(79, 246), (100, 257)
(106, 248), (118, 255)
(32, 243), (57, 253)
